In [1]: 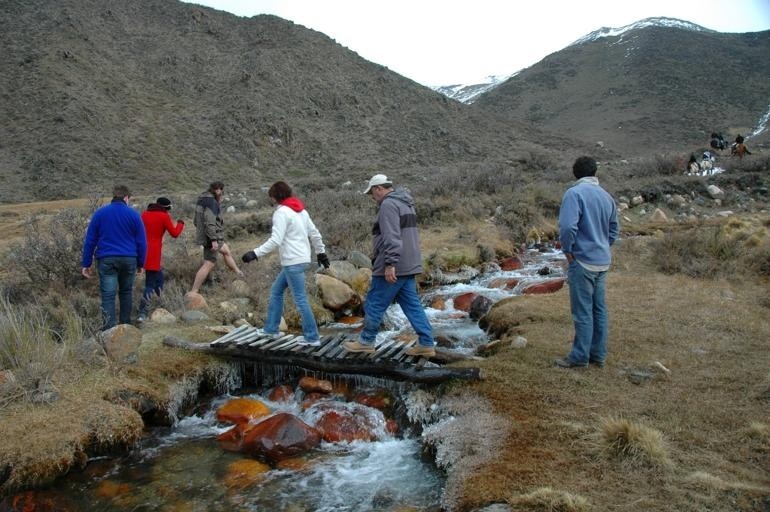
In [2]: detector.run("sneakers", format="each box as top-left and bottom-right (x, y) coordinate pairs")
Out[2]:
(406, 346), (435, 357)
(554, 356), (605, 369)
(298, 340), (321, 346)
(257, 332), (280, 340)
(343, 342), (375, 353)
(137, 314), (149, 320)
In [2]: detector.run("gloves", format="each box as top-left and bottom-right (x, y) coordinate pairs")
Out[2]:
(317, 253), (330, 269)
(242, 251), (257, 262)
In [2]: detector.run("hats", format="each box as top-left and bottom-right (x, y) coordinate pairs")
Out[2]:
(363, 174), (392, 194)
(157, 197), (174, 209)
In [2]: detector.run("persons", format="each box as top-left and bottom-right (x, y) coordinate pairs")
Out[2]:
(82, 184), (184, 331)
(190, 181), (244, 292)
(689, 128), (744, 163)
(554, 157), (619, 368)
(242, 182), (330, 346)
(343, 174), (435, 356)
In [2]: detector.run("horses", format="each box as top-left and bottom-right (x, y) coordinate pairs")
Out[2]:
(690, 156), (716, 177)
(730, 142), (752, 159)
(710, 138), (728, 151)
